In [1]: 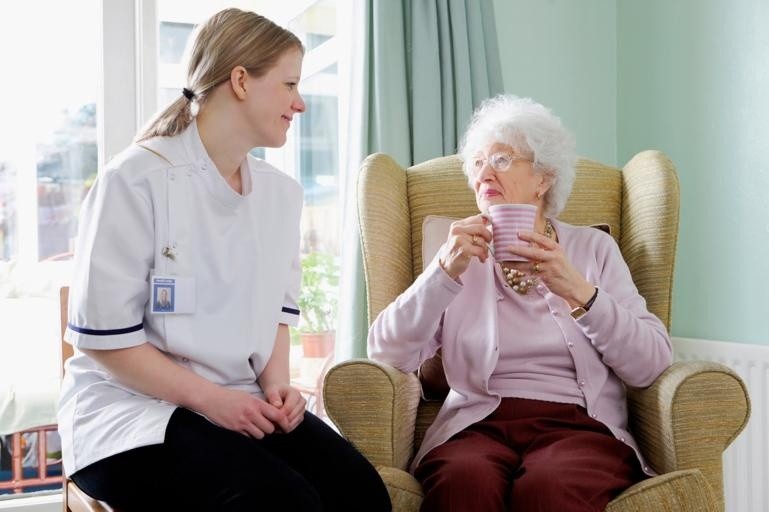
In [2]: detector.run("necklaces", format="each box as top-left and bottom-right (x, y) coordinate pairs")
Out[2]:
(494, 217), (551, 297)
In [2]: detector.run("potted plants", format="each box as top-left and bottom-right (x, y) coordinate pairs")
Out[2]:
(295, 248), (341, 359)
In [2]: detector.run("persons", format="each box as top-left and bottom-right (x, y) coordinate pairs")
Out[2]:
(157, 288), (170, 312)
(53, 5), (396, 511)
(365, 92), (676, 511)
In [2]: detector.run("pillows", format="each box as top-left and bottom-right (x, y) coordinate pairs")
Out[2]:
(417, 213), (613, 402)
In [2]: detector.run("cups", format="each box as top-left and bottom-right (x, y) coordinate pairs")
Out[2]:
(480, 203), (539, 262)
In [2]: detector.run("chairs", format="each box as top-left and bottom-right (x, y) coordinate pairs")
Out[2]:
(59, 284), (109, 512)
(286, 350), (335, 420)
(319, 149), (752, 511)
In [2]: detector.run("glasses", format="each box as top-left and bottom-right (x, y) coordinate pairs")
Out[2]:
(467, 150), (535, 175)
